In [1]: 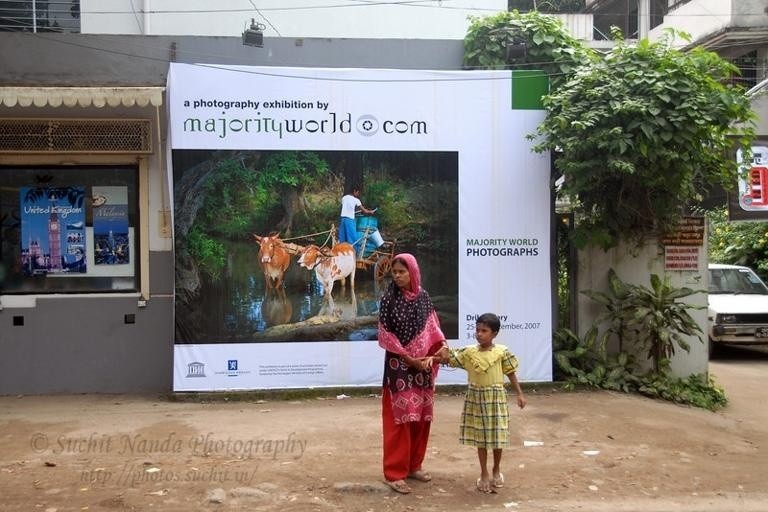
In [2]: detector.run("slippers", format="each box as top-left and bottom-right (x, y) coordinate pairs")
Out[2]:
(476, 477), (492, 493)
(492, 472), (505, 489)
(382, 478), (412, 494)
(407, 467), (432, 482)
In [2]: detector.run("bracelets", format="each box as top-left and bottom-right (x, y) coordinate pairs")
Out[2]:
(442, 345), (448, 349)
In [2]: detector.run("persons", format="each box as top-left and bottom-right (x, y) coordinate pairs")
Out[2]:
(377, 253), (449, 494)
(421, 312), (527, 492)
(337, 185), (375, 255)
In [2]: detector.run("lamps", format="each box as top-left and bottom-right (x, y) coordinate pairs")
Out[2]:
(241, 18), (266, 49)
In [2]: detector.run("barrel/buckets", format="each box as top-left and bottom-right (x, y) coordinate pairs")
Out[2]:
(355, 215), (378, 257)
(368, 230), (384, 247)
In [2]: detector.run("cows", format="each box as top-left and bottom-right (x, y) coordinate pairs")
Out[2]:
(295, 240), (358, 317)
(251, 231), (291, 299)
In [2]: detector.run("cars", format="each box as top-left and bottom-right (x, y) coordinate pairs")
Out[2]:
(708, 263), (768, 359)
